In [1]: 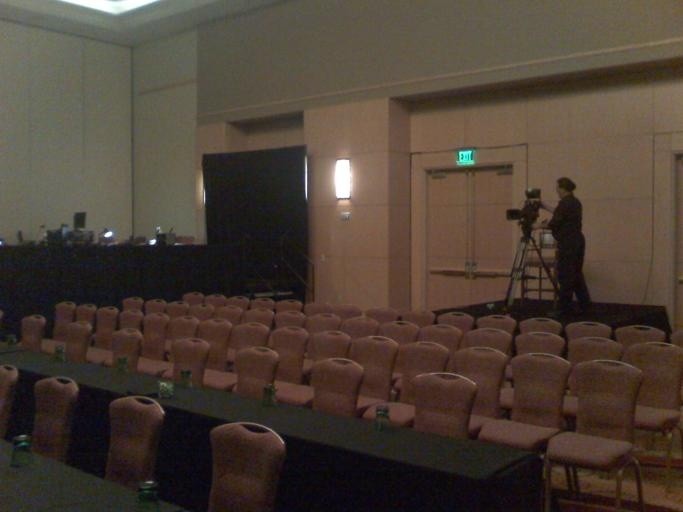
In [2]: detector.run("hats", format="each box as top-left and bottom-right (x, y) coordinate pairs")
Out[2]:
(555, 177), (577, 192)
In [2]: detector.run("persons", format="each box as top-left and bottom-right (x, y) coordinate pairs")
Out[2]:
(530, 177), (594, 323)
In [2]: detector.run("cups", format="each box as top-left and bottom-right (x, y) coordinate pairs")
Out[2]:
(262, 384), (278, 405)
(117, 354), (128, 373)
(137, 479), (160, 511)
(11, 435), (34, 467)
(6, 334), (18, 350)
(53, 344), (65, 361)
(178, 367), (193, 389)
(374, 406), (392, 434)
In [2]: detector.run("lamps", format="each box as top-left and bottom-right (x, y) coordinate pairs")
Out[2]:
(334, 159), (352, 199)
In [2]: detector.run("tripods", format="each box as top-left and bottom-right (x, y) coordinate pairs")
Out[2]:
(503, 224), (560, 311)
